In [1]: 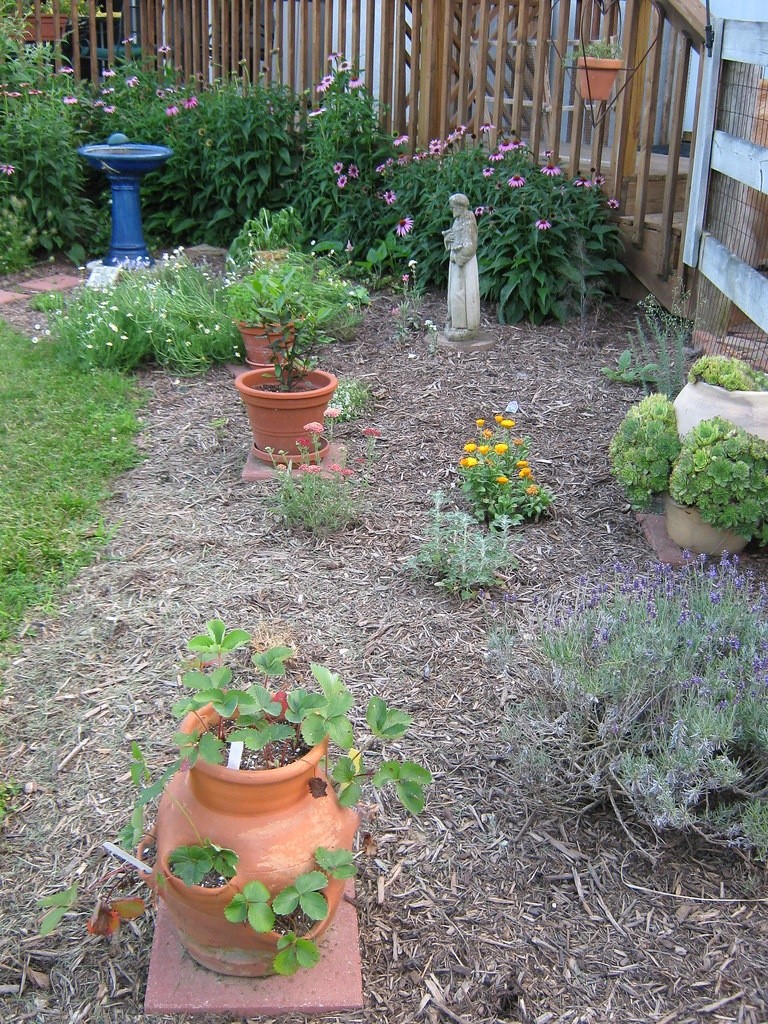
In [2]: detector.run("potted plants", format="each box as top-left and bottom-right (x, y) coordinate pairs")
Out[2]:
(605, 353), (768, 569)
(35, 617), (433, 981)
(224, 203), (342, 471)
(0, 0), (104, 43)
(560, 41), (624, 101)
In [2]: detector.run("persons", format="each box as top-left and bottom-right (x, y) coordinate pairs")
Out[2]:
(441, 193), (481, 331)
